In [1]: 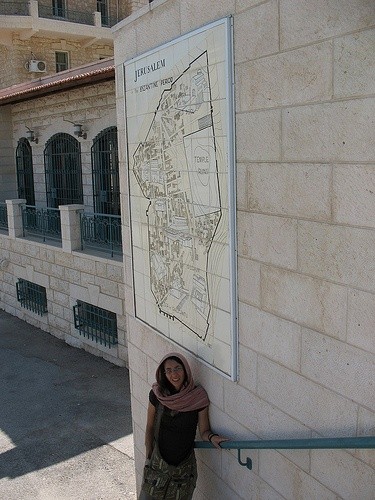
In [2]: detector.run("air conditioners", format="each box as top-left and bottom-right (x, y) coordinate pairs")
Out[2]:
(27, 60), (47, 73)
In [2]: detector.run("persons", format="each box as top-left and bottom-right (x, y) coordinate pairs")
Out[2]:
(136, 351), (232, 500)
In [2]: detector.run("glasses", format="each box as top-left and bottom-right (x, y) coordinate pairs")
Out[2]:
(163, 367), (183, 373)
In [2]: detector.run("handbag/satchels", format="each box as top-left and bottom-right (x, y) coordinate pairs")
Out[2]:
(141, 463), (188, 500)
